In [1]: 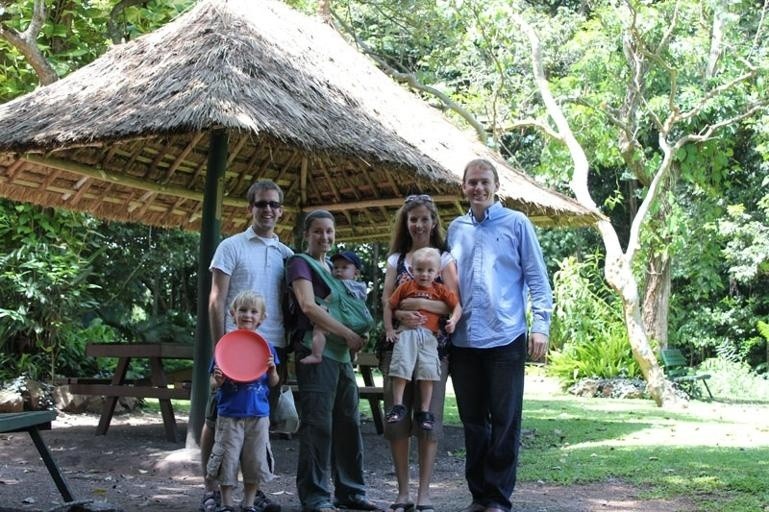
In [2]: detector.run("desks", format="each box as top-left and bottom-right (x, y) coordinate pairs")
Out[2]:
(352, 352), (386, 437)
(85, 338), (194, 444)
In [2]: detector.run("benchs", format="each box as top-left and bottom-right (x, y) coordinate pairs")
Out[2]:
(66, 371), (193, 443)
(286, 383), (384, 436)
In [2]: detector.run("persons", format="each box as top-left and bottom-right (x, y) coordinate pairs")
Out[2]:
(442, 159), (555, 511)
(382, 244), (463, 431)
(301, 247), (374, 366)
(206, 288), (281, 510)
(378, 193), (463, 512)
(201, 179), (297, 512)
(286, 210), (385, 512)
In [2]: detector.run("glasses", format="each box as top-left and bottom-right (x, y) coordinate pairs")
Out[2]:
(252, 200), (282, 209)
(404, 194), (433, 203)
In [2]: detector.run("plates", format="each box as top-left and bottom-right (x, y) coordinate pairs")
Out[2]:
(215, 329), (272, 383)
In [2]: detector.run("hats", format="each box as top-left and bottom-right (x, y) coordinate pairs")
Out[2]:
(329, 250), (360, 266)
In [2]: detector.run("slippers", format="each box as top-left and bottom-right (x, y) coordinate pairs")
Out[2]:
(333, 494), (380, 511)
(312, 500), (340, 512)
(387, 502), (437, 512)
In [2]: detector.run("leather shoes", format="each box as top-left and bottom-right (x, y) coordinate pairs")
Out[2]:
(457, 501), (511, 512)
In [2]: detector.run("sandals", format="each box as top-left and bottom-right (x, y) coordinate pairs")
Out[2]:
(198, 489), (237, 512)
(238, 488), (282, 512)
(384, 404), (408, 425)
(417, 407), (436, 432)
